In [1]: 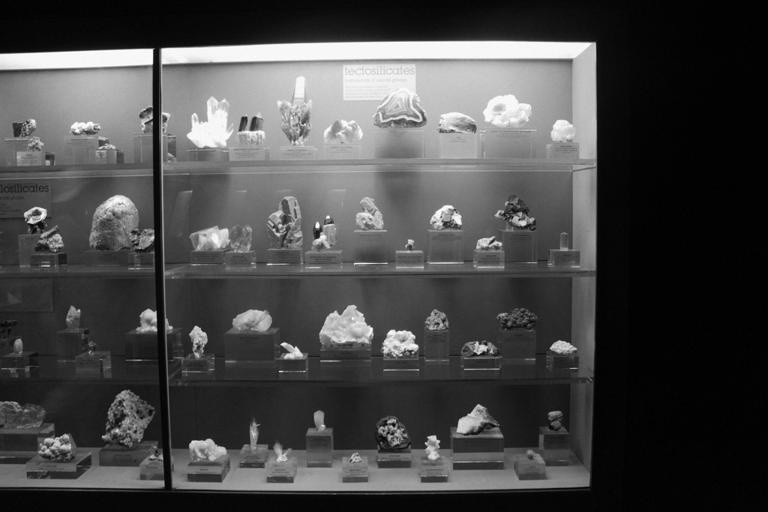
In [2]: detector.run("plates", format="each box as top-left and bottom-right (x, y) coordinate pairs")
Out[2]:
(0, 40), (599, 495)
